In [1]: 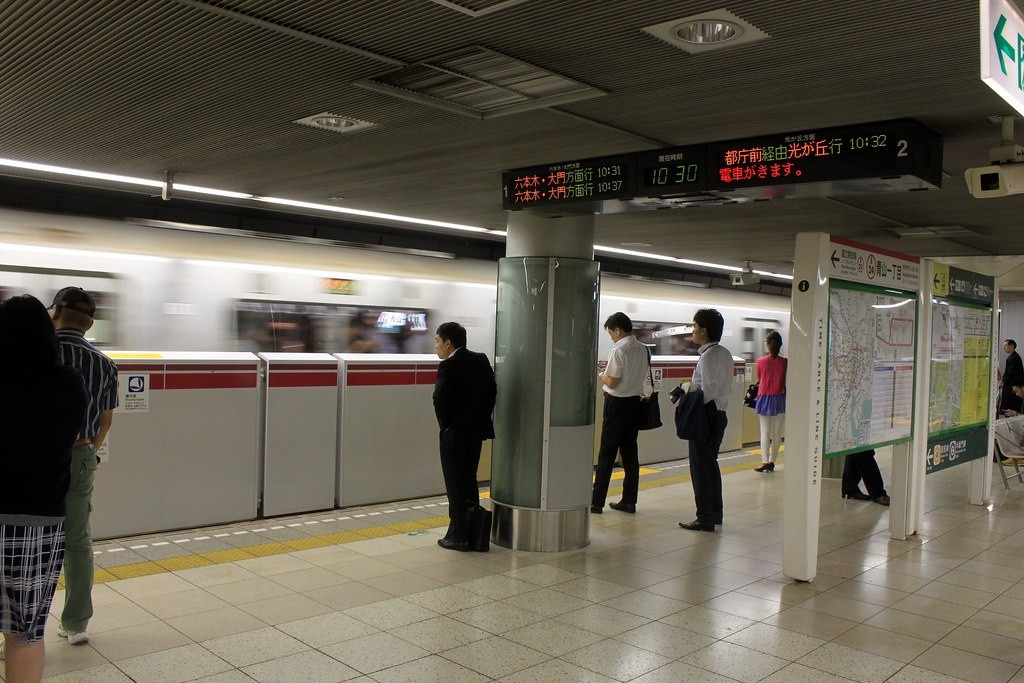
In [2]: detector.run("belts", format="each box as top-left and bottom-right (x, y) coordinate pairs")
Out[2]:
(74, 437), (91, 447)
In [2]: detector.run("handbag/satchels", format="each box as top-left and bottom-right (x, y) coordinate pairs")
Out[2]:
(467, 505), (493, 552)
(637, 391), (662, 431)
(743, 381), (760, 409)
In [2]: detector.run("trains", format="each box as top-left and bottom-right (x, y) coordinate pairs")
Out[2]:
(0, 164), (786, 542)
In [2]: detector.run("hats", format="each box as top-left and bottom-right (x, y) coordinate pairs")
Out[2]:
(47, 287), (96, 318)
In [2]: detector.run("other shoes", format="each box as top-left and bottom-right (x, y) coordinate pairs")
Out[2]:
(841, 488), (870, 500)
(873, 492), (890, 506)
(1002, 456), (1024, 466)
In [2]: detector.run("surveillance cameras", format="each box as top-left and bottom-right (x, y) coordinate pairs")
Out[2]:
(965, 161), (1024, 198)
(729, 273), (760, 285)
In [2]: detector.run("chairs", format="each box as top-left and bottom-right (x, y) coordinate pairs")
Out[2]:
(993, 415), (1024, 490)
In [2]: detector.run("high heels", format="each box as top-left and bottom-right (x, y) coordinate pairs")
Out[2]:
(755, 460), (774, 473)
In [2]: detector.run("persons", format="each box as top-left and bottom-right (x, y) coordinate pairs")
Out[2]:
(841, 449), (890, 505)
(679, 309), (735, 533)
(753, 331), (787, 472)
(590, 312), (651, 514)
(993, 339), (1024, 466)
(0, 293), (90, 683)
(432, 322), (497, 551)
(0, 286), (120, 659)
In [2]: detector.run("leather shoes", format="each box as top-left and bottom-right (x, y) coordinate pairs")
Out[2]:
(716, 517), (722, 525)
(438, 534), (468, 552)
(679, 518), (715, 532)
(608, 499), (636, 513)
(591, 504), (602, 515)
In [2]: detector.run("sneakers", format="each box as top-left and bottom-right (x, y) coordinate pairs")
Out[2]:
(58, 619), (89, 644)
(0, 640), (7, 660)
